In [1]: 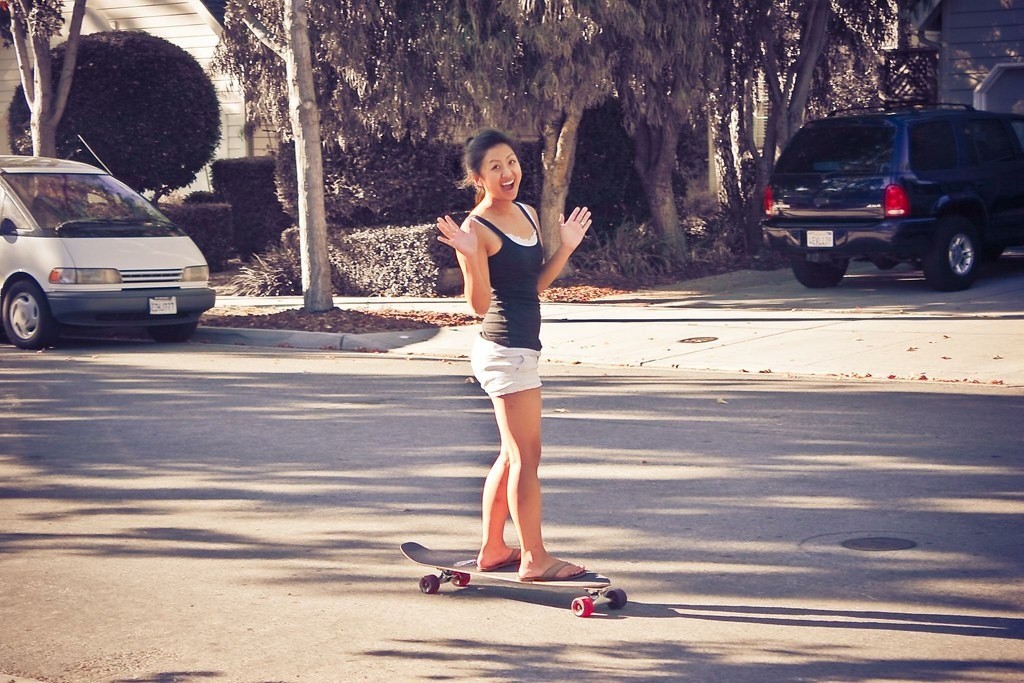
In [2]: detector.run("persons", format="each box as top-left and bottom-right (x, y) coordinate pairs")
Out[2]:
(435, 127), (593, 581)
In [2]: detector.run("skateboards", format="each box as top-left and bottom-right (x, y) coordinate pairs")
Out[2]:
(399, 540), (628, 617)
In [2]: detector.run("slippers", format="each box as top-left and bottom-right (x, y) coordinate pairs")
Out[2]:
(477, 548), (520, 571)
(518, 561), (586, 581)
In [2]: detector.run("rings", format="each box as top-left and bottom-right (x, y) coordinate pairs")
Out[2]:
(451, 240), (454, 244)
(452, 234), (455, 237)
(455, 229), (458, 232)
(580, 221), (584, 226)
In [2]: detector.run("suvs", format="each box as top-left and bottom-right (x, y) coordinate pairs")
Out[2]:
(761, 103), (1024, 293)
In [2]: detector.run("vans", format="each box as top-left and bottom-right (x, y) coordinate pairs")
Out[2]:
(0, 156), (217, 350)
